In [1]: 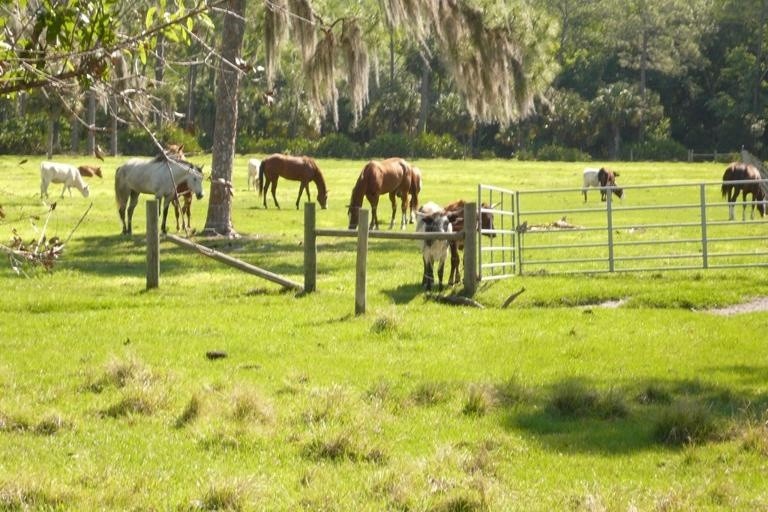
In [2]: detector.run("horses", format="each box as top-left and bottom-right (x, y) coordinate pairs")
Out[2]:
(345, 156), (422, 229)
(720, 163), (766, 221)
(114, 142), (205, 241)
(258, 153), (329, 209)
(246, 158), (261, 192)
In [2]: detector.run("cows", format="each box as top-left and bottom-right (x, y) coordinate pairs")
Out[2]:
(580, 167), (624, 204)
(411, 198), (503, 298)
(39, 160), (90, 200)
(78, 165), (103, 179)
